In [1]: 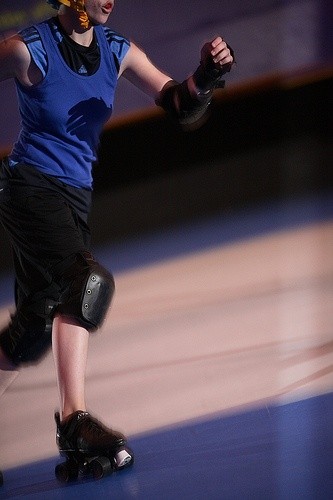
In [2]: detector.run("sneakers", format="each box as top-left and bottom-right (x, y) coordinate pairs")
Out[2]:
(55, 411), (133, 484)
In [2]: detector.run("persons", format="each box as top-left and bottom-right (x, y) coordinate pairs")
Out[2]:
(0, 0), (236, 483)
